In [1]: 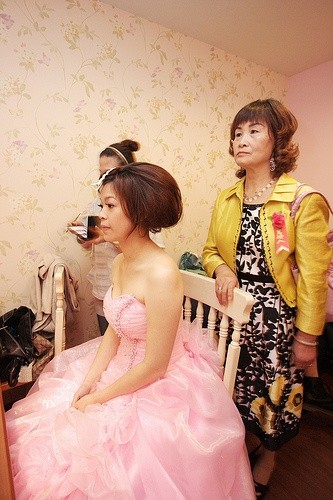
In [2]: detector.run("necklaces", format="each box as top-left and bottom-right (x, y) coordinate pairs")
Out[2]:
(242, 176), (277, 202)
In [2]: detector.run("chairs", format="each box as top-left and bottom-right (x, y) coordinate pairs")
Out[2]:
(0, 265), (66, 391)
(177, 268), (254, 400)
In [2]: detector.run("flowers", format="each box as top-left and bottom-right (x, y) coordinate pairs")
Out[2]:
(271, 211), (286, 229)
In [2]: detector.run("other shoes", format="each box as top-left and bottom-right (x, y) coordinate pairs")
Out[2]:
(251, 451), (275, 499)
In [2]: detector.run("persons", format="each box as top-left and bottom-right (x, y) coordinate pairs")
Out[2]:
(70, 138), (166, 337)
(4, 160), (258, 500)
(200, 98), (331, 500)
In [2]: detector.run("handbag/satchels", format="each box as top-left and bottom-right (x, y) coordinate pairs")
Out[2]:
(0, 306), (37, 388)
(290, 182), (333, 324)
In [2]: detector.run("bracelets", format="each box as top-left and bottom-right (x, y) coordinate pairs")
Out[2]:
(292, 335), (318, 347)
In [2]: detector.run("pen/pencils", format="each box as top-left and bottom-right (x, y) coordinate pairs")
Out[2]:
(65, 213), (80, 232)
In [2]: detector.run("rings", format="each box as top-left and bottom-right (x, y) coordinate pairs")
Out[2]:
(218, 287), (223, 290)
